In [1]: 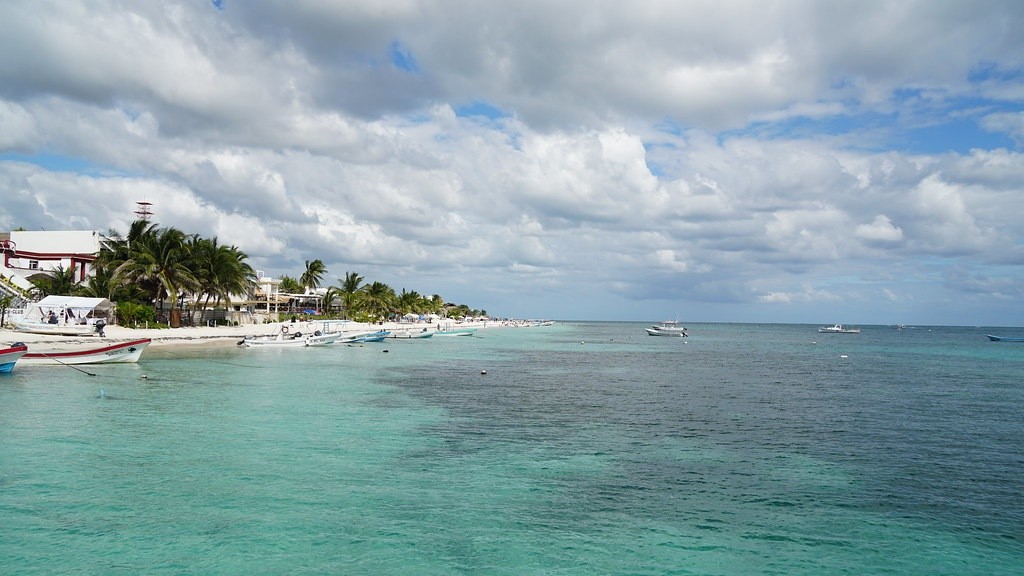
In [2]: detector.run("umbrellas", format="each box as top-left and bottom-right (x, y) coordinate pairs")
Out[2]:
(404, 313), (489, 323)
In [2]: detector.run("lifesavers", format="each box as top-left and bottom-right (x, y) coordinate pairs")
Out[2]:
(281, 326), (288, 333)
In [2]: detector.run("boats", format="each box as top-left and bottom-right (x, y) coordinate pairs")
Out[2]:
(985, 332), (1024, 343)
(0, 342), (28, 373)
(349, 328), (390, 342)
(818, 325), (860, 335)
(15, 338), (151, 363)
(433, 322), (478, 337)
(410, 328), (435, 339)
(503, 317), (553, 328)
(898, 324), (907, 331)
(237, 324), (342, 346)
(644, 320), (688, 337)
(12, 293), (114, 334)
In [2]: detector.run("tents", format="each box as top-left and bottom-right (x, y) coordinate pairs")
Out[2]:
(21, 294), (115, 326)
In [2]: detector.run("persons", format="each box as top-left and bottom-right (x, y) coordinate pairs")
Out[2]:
(47, 309), (58, 324)
(59, 310), (64, 318)
